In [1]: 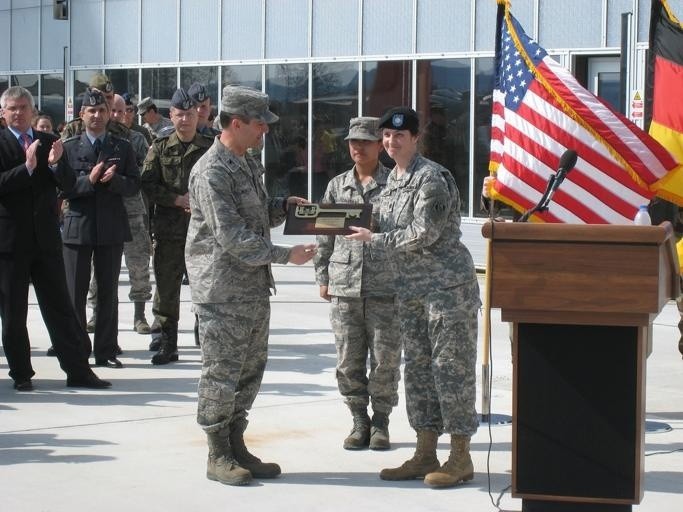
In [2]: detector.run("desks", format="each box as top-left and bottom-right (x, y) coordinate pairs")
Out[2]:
(482, 217), (683, 512)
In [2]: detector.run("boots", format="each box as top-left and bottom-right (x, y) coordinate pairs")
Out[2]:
(117, 346), (121, 355)
(424, 434), (473, 488)
(230, 418), (281, 478)
(47, 346), (55, 356)
(369, 412), (391, 451)
(380, 430), (440, 479)
(87, 308), (97, 332)
(206, 427), (252, 486)
(343, 408), (371, 451)
(134, 302), (151, 334)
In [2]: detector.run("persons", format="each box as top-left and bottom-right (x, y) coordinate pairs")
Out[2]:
(344, 105), (482, 489)
(86, 94), (152, 337)
(159, 82), (221, 139)
(184, 82), (318, 486)
(136, 97), (173, 137)
(312, 117), (403, 449)
(47, 87), (141, 369)
(138, 85), (213, 365)
(35, 114), (53, 134)
(61, 74), (132, 144)
(110, 94), (124, 123)
(124, 93), (153, 143)
(0, 85), (113, 392)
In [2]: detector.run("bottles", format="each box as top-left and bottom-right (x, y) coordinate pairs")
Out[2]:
(635, 205), (651, 226)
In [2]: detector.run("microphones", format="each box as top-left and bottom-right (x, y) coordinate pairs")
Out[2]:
(542, 148), (577, 208)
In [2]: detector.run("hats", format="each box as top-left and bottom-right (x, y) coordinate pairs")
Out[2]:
(83, 87), (106, 105)
(89, 74), (113, 94)
(222, 84), (280, 124)
(137, 97), (154, 115)
(188, 82), (209, 102)
(379, 106), (419, 131)
(344, 117), (382, 141)
(122, 92), (135, 106)
(172, 88), (193, 110)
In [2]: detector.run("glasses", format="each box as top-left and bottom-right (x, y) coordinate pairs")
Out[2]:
(126, 107), (135, 112)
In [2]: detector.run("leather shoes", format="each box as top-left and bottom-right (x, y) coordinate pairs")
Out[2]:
(96, 356), (122, 368)
(152, 349), (178, 365)
(13, 378), (32, 390)
(149, 338), (161, 351)
(67, 369), (112, 387)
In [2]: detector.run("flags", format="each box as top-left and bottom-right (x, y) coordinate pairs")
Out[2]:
(480, 1), (676, 224)
(636, 0), (682, 275)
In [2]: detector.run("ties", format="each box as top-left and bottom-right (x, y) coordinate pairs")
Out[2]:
(93, 138), (102, 157)
(21, 133), (30, 157)
